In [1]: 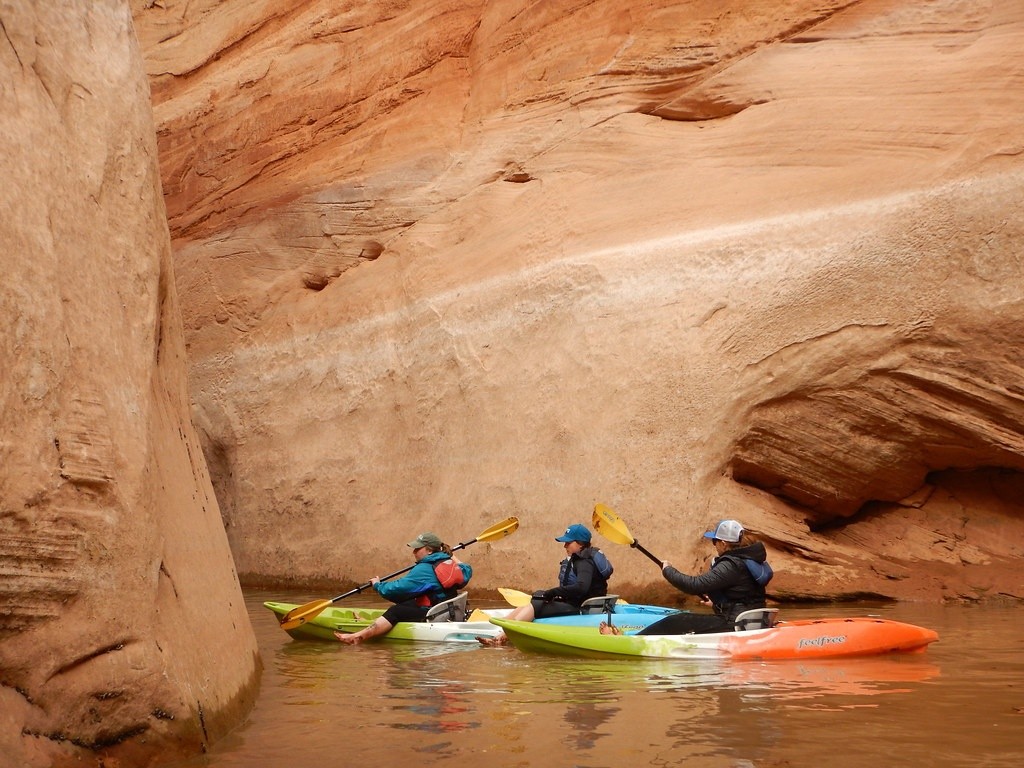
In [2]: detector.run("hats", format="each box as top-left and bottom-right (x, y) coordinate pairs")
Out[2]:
(555, 524), (592, 542)
(704, 520), (745, 542)
(407, 532), (443, 551)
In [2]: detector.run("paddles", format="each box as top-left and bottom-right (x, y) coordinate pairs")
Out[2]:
(280, 516), (520, 631)
(496, 587), (629, 607)
(592, 503), (710, 602)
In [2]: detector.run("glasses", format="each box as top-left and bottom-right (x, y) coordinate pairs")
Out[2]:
(711, 538), (722, 546)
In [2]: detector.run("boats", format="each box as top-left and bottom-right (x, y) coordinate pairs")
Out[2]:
(489, 604), (940, 658)
(264, 599), (628, 643)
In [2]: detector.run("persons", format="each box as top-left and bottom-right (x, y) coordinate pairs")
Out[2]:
(599, 520), (767, 636)
(475, 524), (608, 645)
(333, 531), (473, 645)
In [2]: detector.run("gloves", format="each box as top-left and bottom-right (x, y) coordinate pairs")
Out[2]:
(543, 588), (555, 603)
